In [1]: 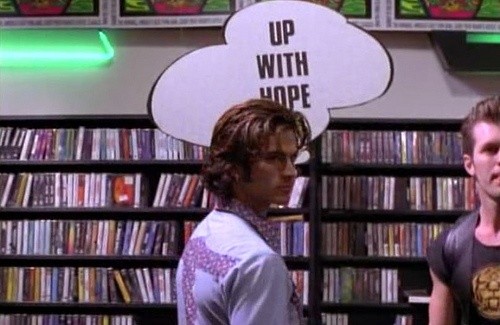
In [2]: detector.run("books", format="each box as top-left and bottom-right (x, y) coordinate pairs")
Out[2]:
(0, 127), (310, 325)
(318, 130), (482, 325)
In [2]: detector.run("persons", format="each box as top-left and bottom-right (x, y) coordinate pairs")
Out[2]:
(176, 98), (310, 325)
(425, 97), (500, 325)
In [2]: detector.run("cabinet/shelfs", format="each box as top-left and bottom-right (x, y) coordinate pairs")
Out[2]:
(0, 114), (500, 325)
